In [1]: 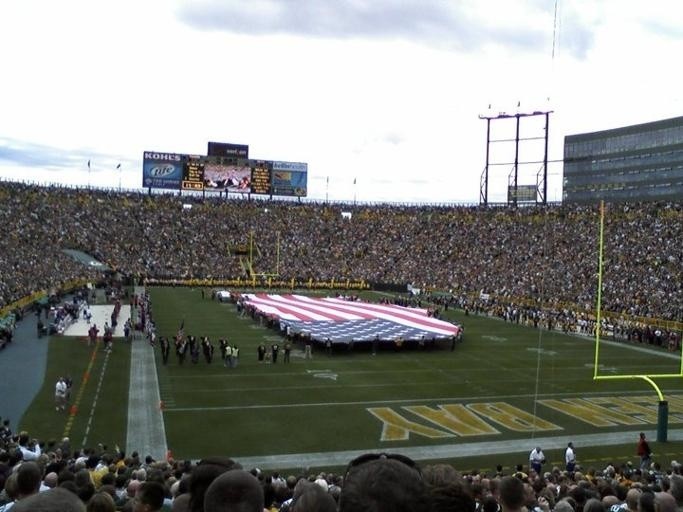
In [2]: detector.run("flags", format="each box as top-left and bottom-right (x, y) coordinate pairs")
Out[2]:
(116, 164), (120, 168)
(86, 160), (90, 168)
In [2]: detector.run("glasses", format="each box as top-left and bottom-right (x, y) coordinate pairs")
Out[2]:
(342, 454), (423, 487)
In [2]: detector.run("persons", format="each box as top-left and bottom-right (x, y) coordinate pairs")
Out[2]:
(1, 178), (682, 368)
(0, 418), (682, 512)
(66, 377), (73, 399)
(204, 163), (250, 191)
(54, 376), (65, 409)
